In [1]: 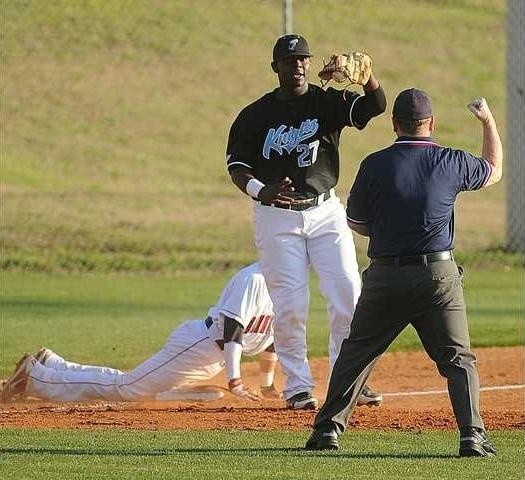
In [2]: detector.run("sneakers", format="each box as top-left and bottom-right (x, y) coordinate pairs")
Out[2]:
(356, 384), (383, 406)
(34, 345), (54, 366)
(305, 424), (341, 449)
(285, 391), (319, 412)
(459, 427), (499, 458)
(0, 352), (37, 402)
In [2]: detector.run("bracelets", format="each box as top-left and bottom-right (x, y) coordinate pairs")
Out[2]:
(245, 177), (266, 199)
(259, 369), (277, 387)
(229, 376), (244, 391)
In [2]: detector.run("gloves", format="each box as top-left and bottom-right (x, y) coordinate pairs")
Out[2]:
(230, 377), (263, 405)
(260, 385), (284, 400)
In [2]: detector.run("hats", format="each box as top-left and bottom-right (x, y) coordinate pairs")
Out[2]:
(272, 34), (314, 62)
(392, 88), (432, 121)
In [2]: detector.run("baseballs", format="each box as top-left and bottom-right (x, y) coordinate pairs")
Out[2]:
(333, 71), (346, 83)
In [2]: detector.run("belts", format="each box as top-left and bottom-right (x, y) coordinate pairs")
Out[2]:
(260, 191), (331, 211)
(371, 250), (452, 267)
(204, 317), (225, 352)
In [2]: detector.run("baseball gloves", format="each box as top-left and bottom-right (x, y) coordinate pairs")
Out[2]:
(318, 51), (372, 85)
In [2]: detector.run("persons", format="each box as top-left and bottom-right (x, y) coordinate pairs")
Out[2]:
(305, 88), (503, 458)
(225, 33), (388, 411)
(0, 261), (283, 403)
